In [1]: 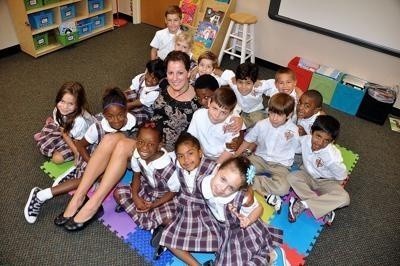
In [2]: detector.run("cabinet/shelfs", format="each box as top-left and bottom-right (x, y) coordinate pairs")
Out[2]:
(5, 1), (113, 58)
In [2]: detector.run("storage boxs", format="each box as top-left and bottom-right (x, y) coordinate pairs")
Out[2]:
(22, 1), (106, 49)
(308, 63), (341, 105)
(286, 55), (320, 91)
(329, 73), (370, 116)
(356, 82), (396, 126)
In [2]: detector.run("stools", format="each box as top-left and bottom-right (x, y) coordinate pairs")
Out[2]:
(217, 11), (258, 65)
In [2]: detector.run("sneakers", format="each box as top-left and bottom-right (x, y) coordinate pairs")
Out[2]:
(24, 187), (44, 224)
(264, 193), (283, 214)
(289, 196), (304, 223)
(51, 166), (79, 188)
(325, 211), (335, 225)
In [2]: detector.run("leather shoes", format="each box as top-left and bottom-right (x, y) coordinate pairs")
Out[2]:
(64, 200), (104, 232)
(150, 223), (166, 247)
(54, 193), (89, 227)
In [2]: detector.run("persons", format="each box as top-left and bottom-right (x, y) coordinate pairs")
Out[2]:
(197, 157), (275, 266)
(54, 50), (241, 233)
(287, 115), (350, 226)
(150, 4), (185, 60)
(112, 50), (323, 233)
(24, 81), (93, 164)
(159, 132), (224, 266)
(24, 87), (139, 223)
